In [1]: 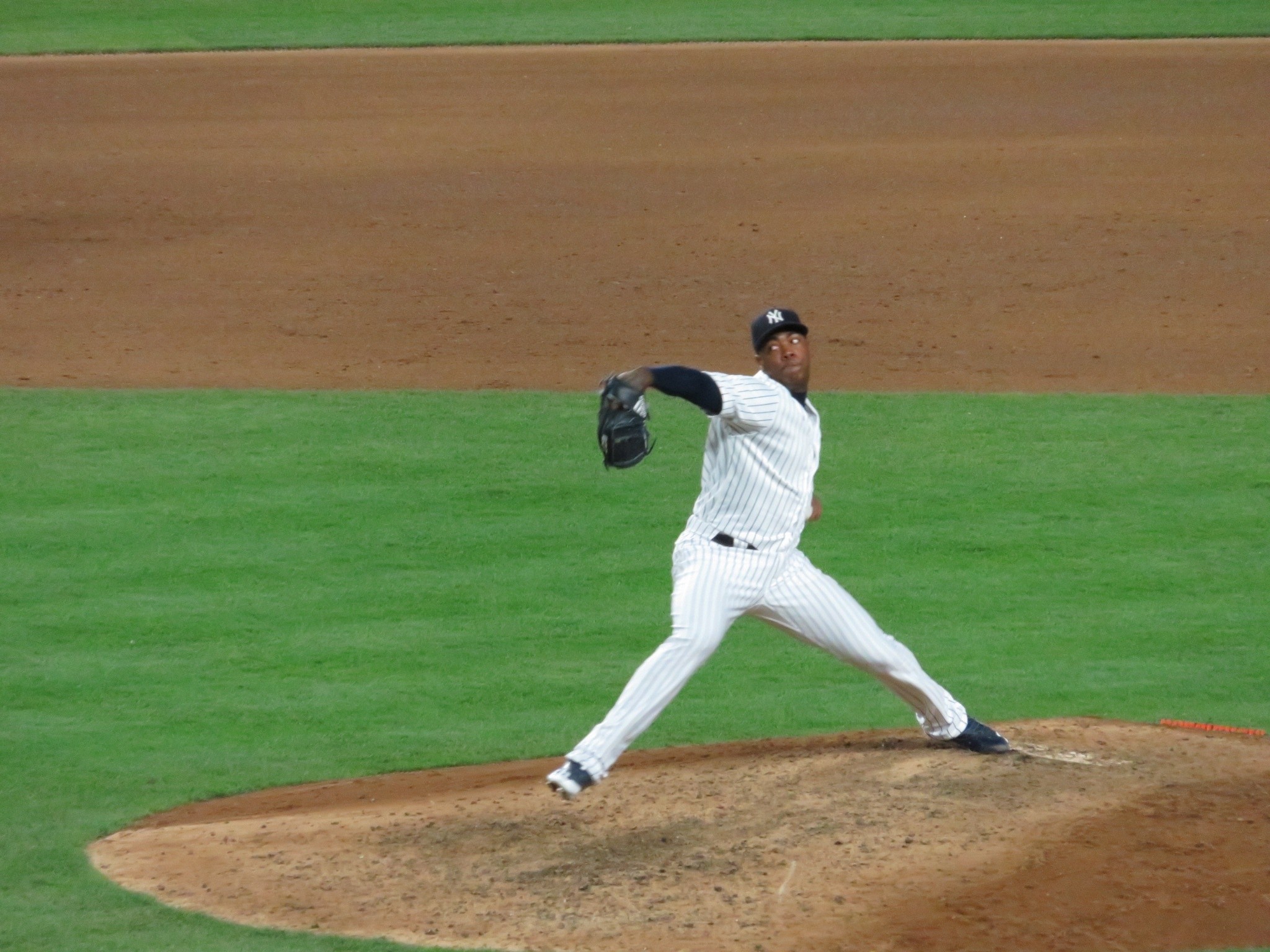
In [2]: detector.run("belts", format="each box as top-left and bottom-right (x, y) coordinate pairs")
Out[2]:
(711, 532), (757, 550)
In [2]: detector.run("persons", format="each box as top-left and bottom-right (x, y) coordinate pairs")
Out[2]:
(544, 308), (1011, 794)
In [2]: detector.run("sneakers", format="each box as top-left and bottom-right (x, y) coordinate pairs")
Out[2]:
(545, 758), (595, 800)
(933, 714), (1011, 756)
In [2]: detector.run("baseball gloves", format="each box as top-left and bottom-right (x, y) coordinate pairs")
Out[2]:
(595, 371), (656, 473)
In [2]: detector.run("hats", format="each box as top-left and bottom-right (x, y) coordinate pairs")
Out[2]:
(750, 306), (809, 355)
